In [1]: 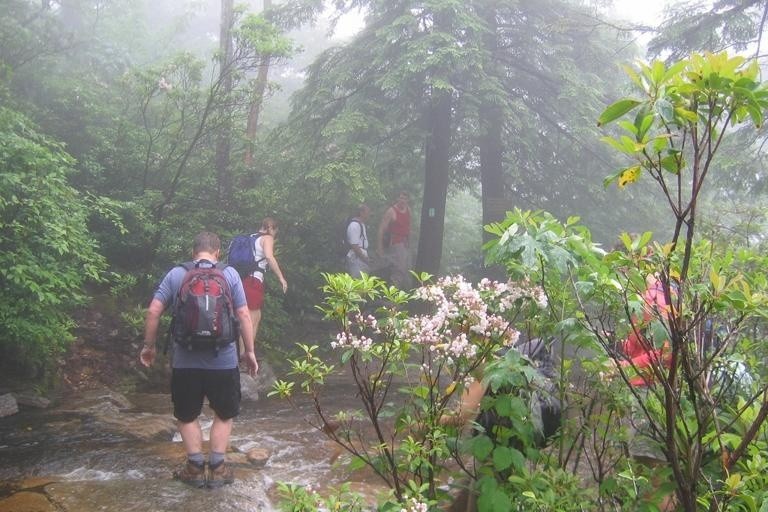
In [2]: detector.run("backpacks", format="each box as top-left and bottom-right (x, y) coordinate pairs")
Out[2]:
(227, 233), (267, 279)
(332, 218), (365, 255)
(170, 260), (240, 351)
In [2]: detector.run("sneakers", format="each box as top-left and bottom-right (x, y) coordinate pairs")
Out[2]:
(206, 460), (234, 487)
(239, 359), (248, 372)
(174, 460), (204, 487)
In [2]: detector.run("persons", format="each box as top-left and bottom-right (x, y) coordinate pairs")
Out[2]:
(345, 205), (370, 279)
(377, 191), (412, 272)
(222, 218), (287, 363)
(140, 232), (258, 488)
(433, 339), (562, 458)
